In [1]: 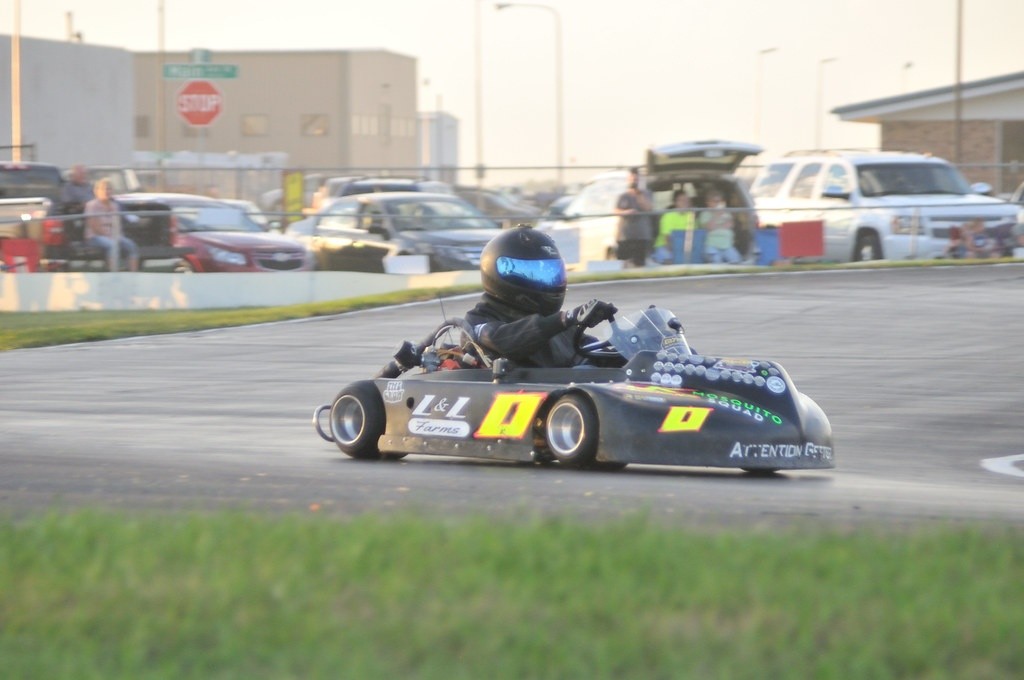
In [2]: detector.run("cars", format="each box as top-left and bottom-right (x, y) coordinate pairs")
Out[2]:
(114, 190), (314, 278)
(322, 171), (550, 234)
(541, 136), (761, 279)
(290, 190), (501, 273)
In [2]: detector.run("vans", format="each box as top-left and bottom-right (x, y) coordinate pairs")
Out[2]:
(1, 157), (85, 266)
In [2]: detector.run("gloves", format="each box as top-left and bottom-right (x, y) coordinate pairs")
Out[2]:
(566, 299), (617, 327)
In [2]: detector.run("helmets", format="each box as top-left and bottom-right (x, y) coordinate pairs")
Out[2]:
(480, 223), (567, 316)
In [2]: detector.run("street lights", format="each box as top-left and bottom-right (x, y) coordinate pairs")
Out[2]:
(494, 0), (566, 191)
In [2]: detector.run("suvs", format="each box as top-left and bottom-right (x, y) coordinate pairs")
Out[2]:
(755, 147), (1024, 261)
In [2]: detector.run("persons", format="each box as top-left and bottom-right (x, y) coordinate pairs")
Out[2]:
(465, 224), (697, 369)
(616, 168), (743, 268)
(41, 161), (140, 271)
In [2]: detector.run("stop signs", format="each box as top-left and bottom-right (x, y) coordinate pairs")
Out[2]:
(176, 78), (223, 126)
